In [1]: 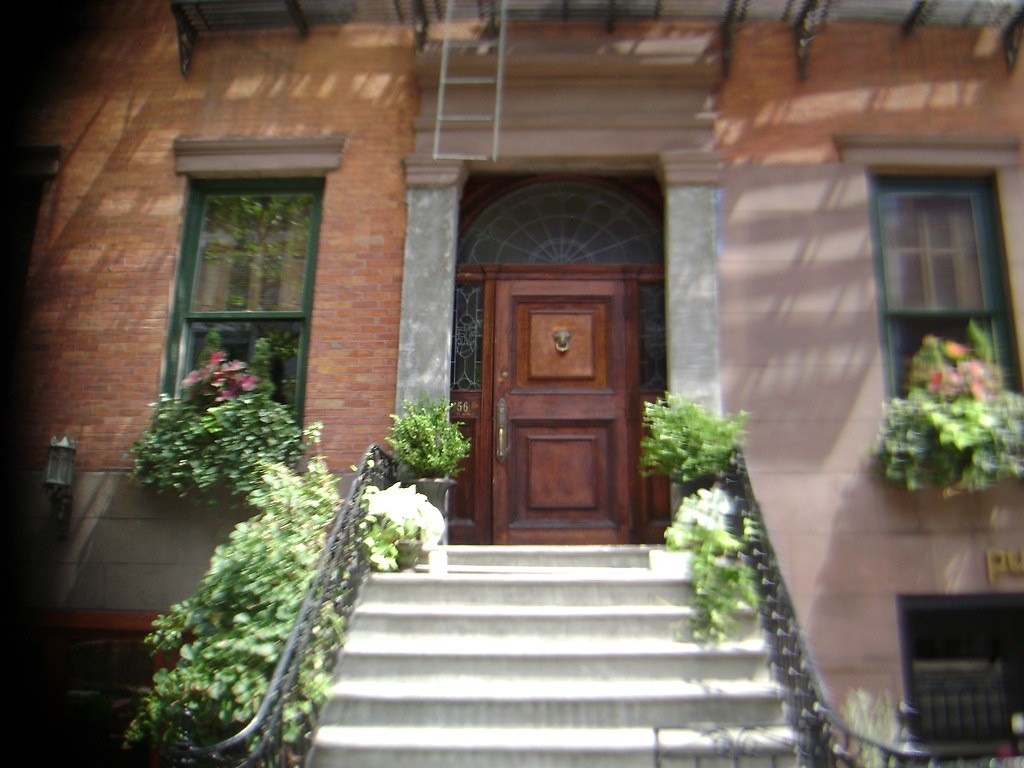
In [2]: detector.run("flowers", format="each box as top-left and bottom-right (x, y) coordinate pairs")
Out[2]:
(120, 326), (307, 512)
(866, 318), (1024, 501)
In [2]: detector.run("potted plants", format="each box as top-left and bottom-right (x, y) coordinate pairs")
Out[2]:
(675, 527), (759, 647)
(358, 482), (446, 573)
(383, 387), (471, 519)
(648, 487), (736, 576)
(637, 389), (754, 521)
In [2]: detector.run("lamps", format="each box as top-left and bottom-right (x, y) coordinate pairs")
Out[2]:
(41, 436), (76, 544)
